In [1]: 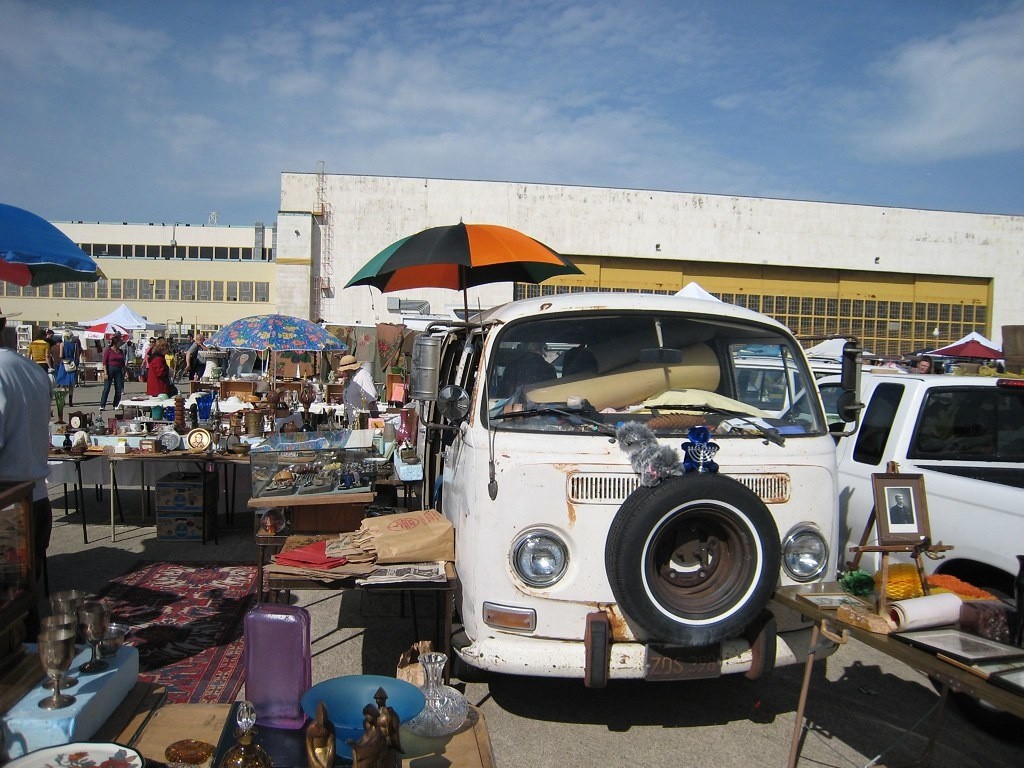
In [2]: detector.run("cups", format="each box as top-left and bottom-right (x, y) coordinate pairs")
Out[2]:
(196, 398), (212, 420)
(211, 368), (222, 379)
(127, 421), (140, 432)
(121, 424), (137, 435)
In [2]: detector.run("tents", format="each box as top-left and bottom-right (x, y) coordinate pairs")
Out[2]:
(674, 282), (723, 303)
(78, 304), (168, 347)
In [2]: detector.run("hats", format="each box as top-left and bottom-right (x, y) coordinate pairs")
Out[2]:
(338, 355), (361, 370)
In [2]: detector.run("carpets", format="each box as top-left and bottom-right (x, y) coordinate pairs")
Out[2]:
(87, 558), (278, 704)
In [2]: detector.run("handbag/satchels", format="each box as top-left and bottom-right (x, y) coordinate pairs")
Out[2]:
(64, 361), (76, 372)
(350, 508), (453, 564)
(168, 380), (178, 398)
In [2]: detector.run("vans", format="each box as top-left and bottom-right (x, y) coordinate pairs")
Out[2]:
(407, 280), (845, 690)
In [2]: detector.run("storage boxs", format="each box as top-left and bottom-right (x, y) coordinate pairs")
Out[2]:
(156, 514), (213, 543)
(219, 380), (255, 403)
(155, 472), (219, 513)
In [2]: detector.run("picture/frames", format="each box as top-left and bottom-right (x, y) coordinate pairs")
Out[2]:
(871, 473), (931, 546)
(887, 627), (1024, 666)
(989, 667), (1024, 695)
(796, 592), (873, 612)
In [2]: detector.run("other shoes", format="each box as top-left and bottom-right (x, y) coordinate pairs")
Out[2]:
(113, 406), (118, 411)
(98, 406), (105, 411)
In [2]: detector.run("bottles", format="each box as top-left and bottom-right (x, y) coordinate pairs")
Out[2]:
(343, 416), (348, 429)
(113, 420), (126, 435)
(342, 462), (376, 488)
(397, 409), (410, 445)
(194, 373), (198, 381)
(304, 371), (306, 382)
(280, 421), (313, 432)
(220, 433), (239, 454)
(63, 434), (72, 452)
(278, 402), (289, 417)
(403, 653), (469, 737)
(166, 406), (174, 420)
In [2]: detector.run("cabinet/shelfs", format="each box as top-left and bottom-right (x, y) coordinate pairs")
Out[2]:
(16, 324), (32, 361)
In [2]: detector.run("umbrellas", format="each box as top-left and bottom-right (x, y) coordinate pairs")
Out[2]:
(344, 217), (584, 338)
(804, 332), (1005, 363)
(204, 312), (349, 390)
(0, 201), (109, 288)
(83, 322), (130, 341)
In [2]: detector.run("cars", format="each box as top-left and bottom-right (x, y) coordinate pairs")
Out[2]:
(719, 332), (1024, 706)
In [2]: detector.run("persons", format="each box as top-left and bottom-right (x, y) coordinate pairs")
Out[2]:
(0, 312), (52, 637)
(890, 493), (915, 524)
(140, 337), (178, 398)
(918, 356), (932, 374)
(99, 335), (134, 410)
(26, 330), (51, 375)
(338, 355), (378, 425)
(95, 339), (102, 353)
(45, 329), (55, 346)
(56, 331), (80, 406)
(179, 334), (208, 381)
(51, 339), (62, 374)
(75, 339), (82, 355)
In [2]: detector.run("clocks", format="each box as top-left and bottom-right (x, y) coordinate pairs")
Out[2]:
(67, 411), (90, 432)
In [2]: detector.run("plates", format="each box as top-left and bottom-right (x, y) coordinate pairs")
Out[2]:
(3, 742), (145, 768)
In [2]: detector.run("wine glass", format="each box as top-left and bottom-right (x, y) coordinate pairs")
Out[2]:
(301, 675), (426, 760)
(212, 434), (220, 453)
(232, 444), (251, 457)
(38, 590), (111, 709)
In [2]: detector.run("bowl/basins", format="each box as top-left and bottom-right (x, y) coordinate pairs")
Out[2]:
(241, 374), (259, 380)
(95, 623), (131, 658)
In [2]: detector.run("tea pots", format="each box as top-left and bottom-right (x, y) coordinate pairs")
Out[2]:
(402, 454), (423, 465)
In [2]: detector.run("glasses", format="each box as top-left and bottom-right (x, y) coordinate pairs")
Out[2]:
(150, 341), (154, 343)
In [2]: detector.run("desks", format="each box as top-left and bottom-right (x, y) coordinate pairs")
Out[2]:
(772, 583), (1024, 768)
(0, 381), (496, 768)
